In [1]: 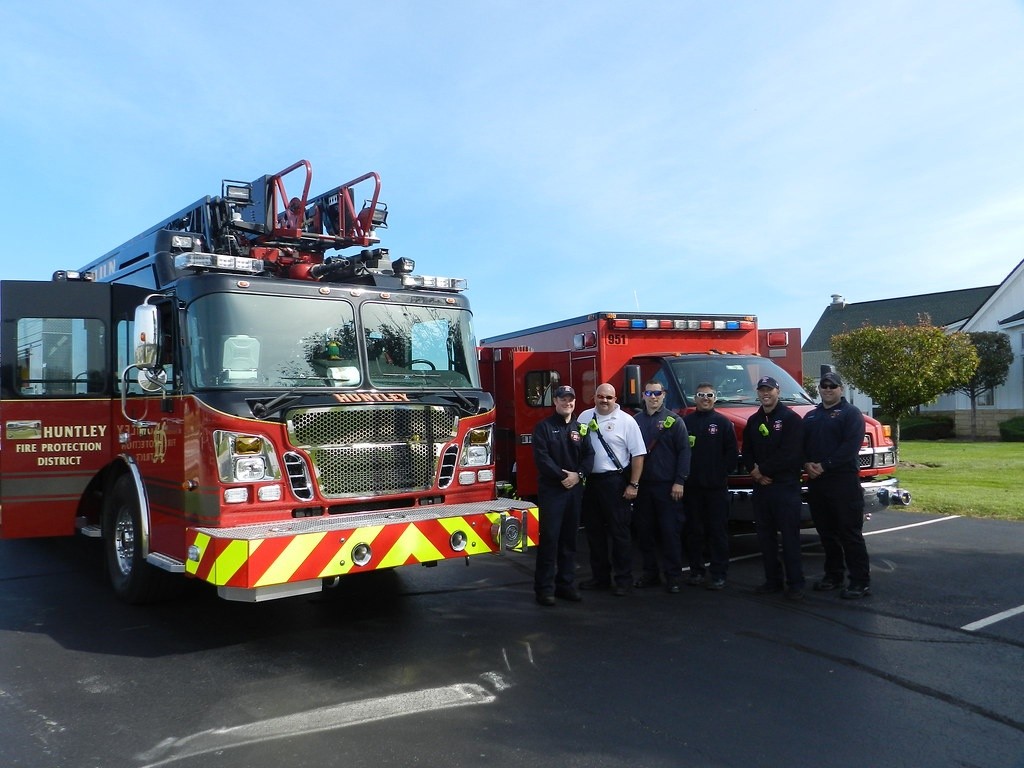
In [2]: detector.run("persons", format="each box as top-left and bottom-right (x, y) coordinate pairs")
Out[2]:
(802, 372), (871, 600)
(531, 385), (595, 606)
(576, 382), (647, 596)
(743, 378), (806, 604)
(682, 383), (739, 590)
(632, 379), (692, 592)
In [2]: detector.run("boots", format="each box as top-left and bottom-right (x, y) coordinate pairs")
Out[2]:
(786, 572), (808, 599)
(756, 571), (784, 593)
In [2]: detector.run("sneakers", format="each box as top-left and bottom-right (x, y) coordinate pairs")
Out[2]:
(812, 572), (844, 590)
(840, 583), (871, 598)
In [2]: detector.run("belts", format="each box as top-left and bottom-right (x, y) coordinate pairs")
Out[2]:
(590, 473), (631, 478)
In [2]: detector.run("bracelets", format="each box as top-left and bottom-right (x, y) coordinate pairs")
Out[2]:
(630, 482), (640, 488)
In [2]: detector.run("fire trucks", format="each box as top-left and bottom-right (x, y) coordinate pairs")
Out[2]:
(478, 310), (914, 529)
(2, 160), (541, 605)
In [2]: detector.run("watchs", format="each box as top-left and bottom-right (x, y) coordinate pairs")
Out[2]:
(579, 471), (584, 480)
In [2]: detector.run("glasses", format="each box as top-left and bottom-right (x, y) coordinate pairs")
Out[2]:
(696, 392), (714, 398)
(820, 383), (841, 389)
(596, 395), (615, 400)
(645, 391), (662, 397)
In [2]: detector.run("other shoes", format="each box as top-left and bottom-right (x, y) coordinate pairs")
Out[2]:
(706, 574), (725, 588)
(666, 576), (681, 592)
(580, 579), (611, 589)
(536, 592), (554, 605)
(555, 585), (582, 600)
(633, 573), (661, 587)
(692, 574), (706, 584)
(614, 584), (633, 595)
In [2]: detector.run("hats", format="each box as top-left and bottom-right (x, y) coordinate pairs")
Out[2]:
(820, 372), (842, 386)
(556, 386), (576, 398)
(758, 377), (779, 390)
